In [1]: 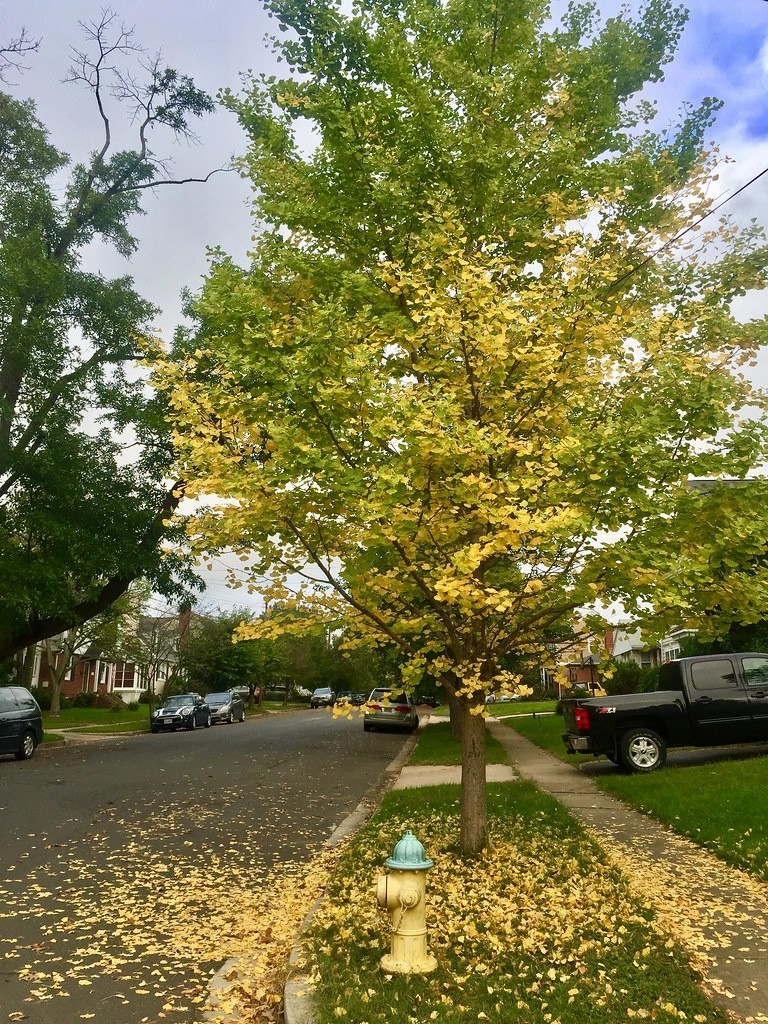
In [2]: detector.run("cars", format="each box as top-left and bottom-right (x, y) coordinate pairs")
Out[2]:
(203, 692), (245, 724)
(485, 692), (520, 702)
(224, 686), (260, 704)
(264, 686), (286, 700)
(150, 694), (211, 733)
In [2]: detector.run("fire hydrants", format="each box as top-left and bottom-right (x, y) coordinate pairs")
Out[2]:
(373, 829), (441, 976)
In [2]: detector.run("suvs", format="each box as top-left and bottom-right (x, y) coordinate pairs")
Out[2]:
(569, 681), (607, 698)
(336, 692), (356, 707)
(354, 693), (367, 705)
(363, 688), (419, 733)
(310, 686), (336, 709)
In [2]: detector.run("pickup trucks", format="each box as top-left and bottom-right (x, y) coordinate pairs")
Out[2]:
(560, 652), (768, 774)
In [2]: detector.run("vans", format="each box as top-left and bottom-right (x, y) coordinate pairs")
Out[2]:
(0, 685), (45, 760)
